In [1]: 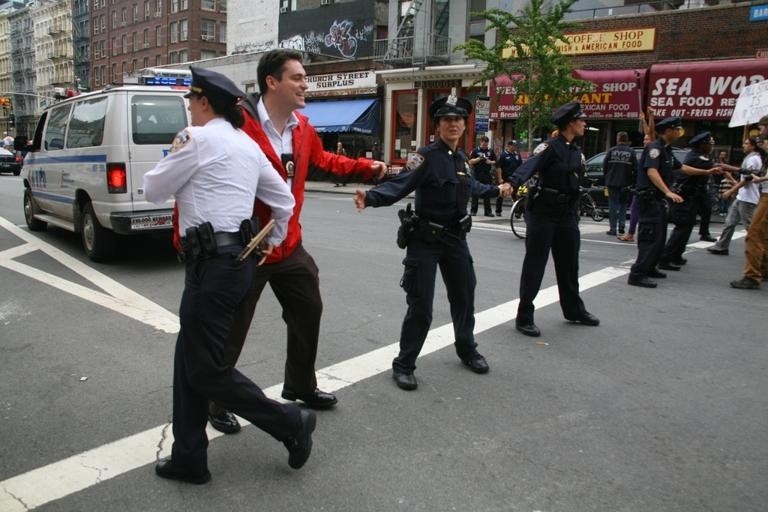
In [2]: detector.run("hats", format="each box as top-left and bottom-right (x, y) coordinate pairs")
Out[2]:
(507, 139), (518, 147)
(656, 115), (683, 132)
(688, 131), (715, 148)
(426, 94), (474, 121)
(181, 63), (248, 104)
(550, 99), (589, 125)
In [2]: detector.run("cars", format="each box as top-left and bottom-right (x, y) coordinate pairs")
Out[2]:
(0, 146), (21, 178)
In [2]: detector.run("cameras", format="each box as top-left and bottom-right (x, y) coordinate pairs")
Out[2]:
(745, 171), (760, 182)
(750, 134), (768, 145)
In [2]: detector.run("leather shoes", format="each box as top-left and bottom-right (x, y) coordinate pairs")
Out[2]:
(567, 310), (601, 328)
(515, 318), (541, 338)
(627, 254), (688, 289)
(457, 352), (490, 375)
(284, 403), (317, 470)
(154, 449), (212, 485)
(280, 382), (339, 412)
(391, 364), (418, 391)
(203, 400), (241, 435)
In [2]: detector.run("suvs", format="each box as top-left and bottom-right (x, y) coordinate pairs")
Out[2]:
(576, 143), (689, 214)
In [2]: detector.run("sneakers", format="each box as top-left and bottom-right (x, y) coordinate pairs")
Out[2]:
(698, 232), (731, 257)
(729, 277), (762, 291)
(605, 228), (636, 242)
(468, 209), (526, 222)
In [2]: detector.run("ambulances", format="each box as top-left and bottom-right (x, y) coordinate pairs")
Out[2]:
(18, 65), (193, 266)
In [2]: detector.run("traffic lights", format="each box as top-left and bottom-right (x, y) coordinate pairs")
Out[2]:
(4, 96), (10, 111)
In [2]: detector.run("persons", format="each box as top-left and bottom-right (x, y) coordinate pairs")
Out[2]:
(467, 134), (496, 218)
(495, 138), (523, 218)
(501, 100), (600, 336)
(332, 140), (346, 187)
(142, 65), (316, 486)
(170, 47), (388, 436)
(353, 95), (511, 392)
(550, 117), (767, 291)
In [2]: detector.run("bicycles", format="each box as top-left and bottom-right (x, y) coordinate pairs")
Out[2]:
(576, 183), (608, 221)
(508, 190), (527, 241)
